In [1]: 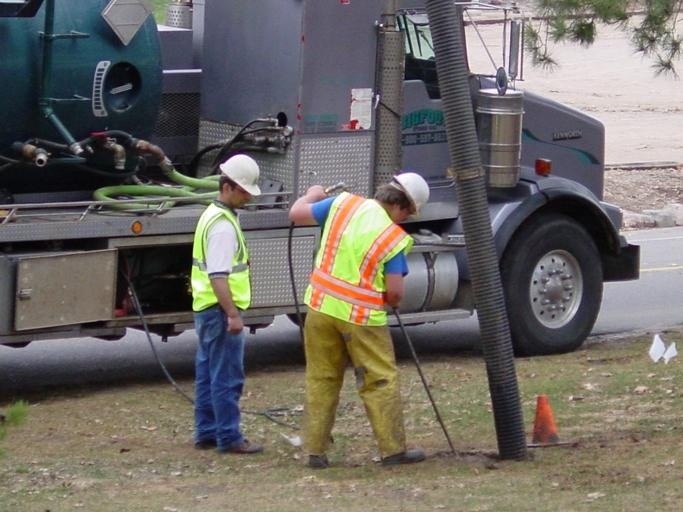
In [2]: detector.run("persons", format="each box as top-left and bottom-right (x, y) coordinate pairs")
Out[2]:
(188, 153), (265, 453)
(286, 170), (430, 467)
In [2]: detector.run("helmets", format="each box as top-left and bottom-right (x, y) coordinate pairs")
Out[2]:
(392, 171), (431, 220)
(218, 153), (263, 197)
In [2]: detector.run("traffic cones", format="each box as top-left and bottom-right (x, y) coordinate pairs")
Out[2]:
(525, 394), (570, 447)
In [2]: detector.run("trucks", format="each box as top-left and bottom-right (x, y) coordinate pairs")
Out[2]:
(0, 0), (641, 357)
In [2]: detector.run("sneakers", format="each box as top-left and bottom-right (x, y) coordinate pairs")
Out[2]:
(195, 435), (217, 447)
(306, 454), (332, 468)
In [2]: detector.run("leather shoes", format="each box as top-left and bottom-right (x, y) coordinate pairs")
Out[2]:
(380, 447), (425, 464)
(226, 438), (267, 454)
